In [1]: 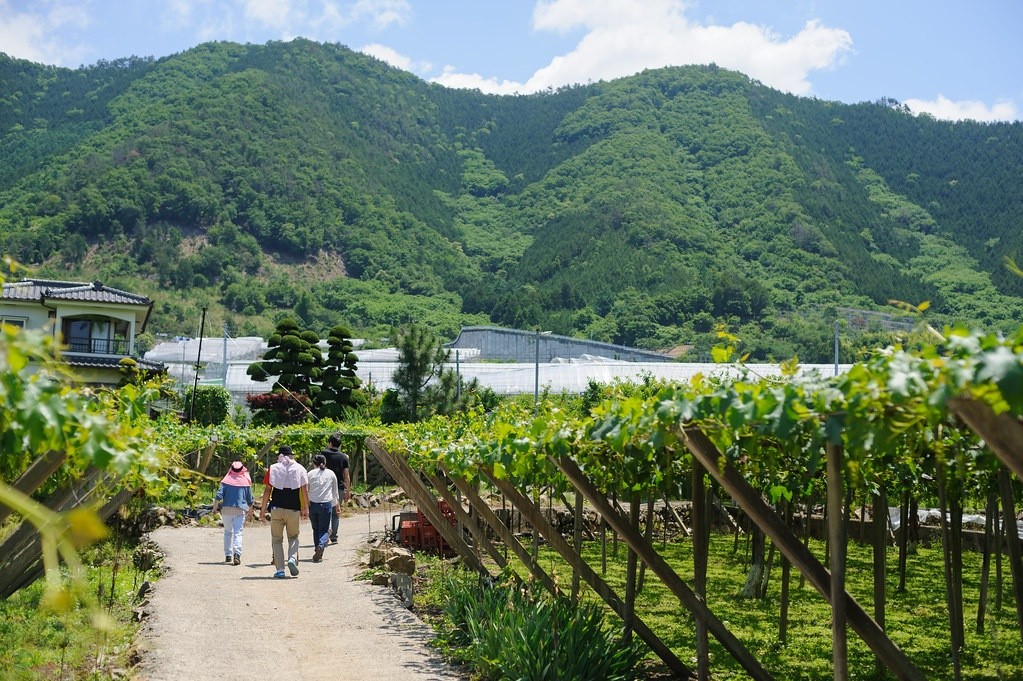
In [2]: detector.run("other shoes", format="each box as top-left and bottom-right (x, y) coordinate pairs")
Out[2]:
(287, 560), (299, 576)
(226, 556), (232, 562)
(313, 545), (323, 562)
(234, 553), (241, 565)
(332, 539), (338, 544)
(274, 571), (285, 578)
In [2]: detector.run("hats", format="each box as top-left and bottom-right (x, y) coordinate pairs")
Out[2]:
(276, 446), (295, 456)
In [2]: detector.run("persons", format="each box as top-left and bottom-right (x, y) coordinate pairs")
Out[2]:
(307, 455), (342, 562)
(320, 433), (351, 546)
(212, 461), (253, 565)
(260, 445), (309, 578)
